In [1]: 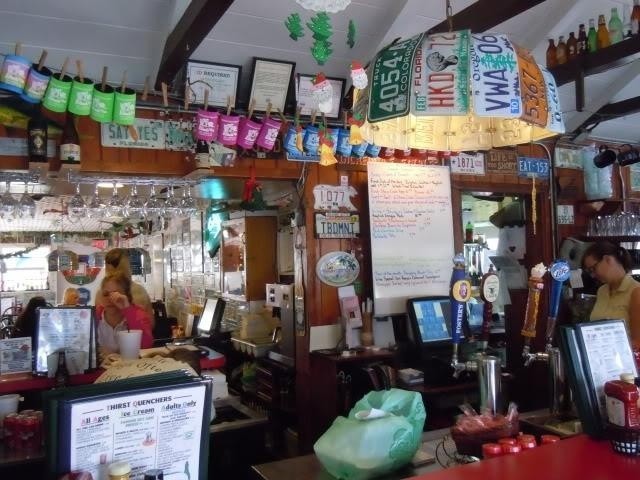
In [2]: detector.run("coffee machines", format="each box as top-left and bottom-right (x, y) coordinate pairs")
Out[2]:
(266, 283), (295, 366)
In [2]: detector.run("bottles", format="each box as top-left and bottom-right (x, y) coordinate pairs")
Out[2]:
(605, 372), (640, 442)
(26, 101), (47, 161)
(465, 221), (474, 243)
(55, 349), (70, 390)
(482, 434), (561, 457)
(549, 349), (572, 415)
(60, 113), (80, 163)
(359, 310), (375, 348)
(479, 357), (502, 416)
(196, 136), (211, 170)
(65, 461), (164, 479)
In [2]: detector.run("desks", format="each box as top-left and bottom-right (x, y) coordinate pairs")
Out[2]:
(34, 367), (212, 479)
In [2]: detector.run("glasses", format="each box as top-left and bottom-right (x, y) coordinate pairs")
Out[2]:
(586, 259), (605, 274)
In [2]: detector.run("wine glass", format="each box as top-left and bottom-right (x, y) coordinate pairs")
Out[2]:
(1, 174), (199, 221)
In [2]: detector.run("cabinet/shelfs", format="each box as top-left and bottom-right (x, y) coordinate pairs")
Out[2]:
(220, 216), (277, 301)
(250, 406), (640, 480)
(306, 344), (517, 454)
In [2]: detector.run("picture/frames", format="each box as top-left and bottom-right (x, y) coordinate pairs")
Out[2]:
(245, 56), (295, 116)
(182, 59), (242, 110)
(294, 72), (347, 121)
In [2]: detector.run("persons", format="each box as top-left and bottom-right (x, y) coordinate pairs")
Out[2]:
(582, 241), (639, 350)
(63, 289), (81, 308)
(96, 249), (153, 327)
(97, 274), (151, 348)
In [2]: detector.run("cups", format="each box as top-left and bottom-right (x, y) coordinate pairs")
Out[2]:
(118, 330), (143, 360)
(2, 54), (456, 158)
(0, 394), (18, 438)
(587, 213), (639, 238)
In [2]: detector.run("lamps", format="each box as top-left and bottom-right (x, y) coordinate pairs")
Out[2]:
(350, 0), (565, 152)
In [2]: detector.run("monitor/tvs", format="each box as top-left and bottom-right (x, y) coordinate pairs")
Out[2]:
(406, 297), (470, 346)
(196, 297), (225, 335)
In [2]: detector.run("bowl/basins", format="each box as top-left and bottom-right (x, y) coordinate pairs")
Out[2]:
(450, 423), (518, 457)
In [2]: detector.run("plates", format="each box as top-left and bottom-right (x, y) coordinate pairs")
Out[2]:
(317, 252), (360, 287)
